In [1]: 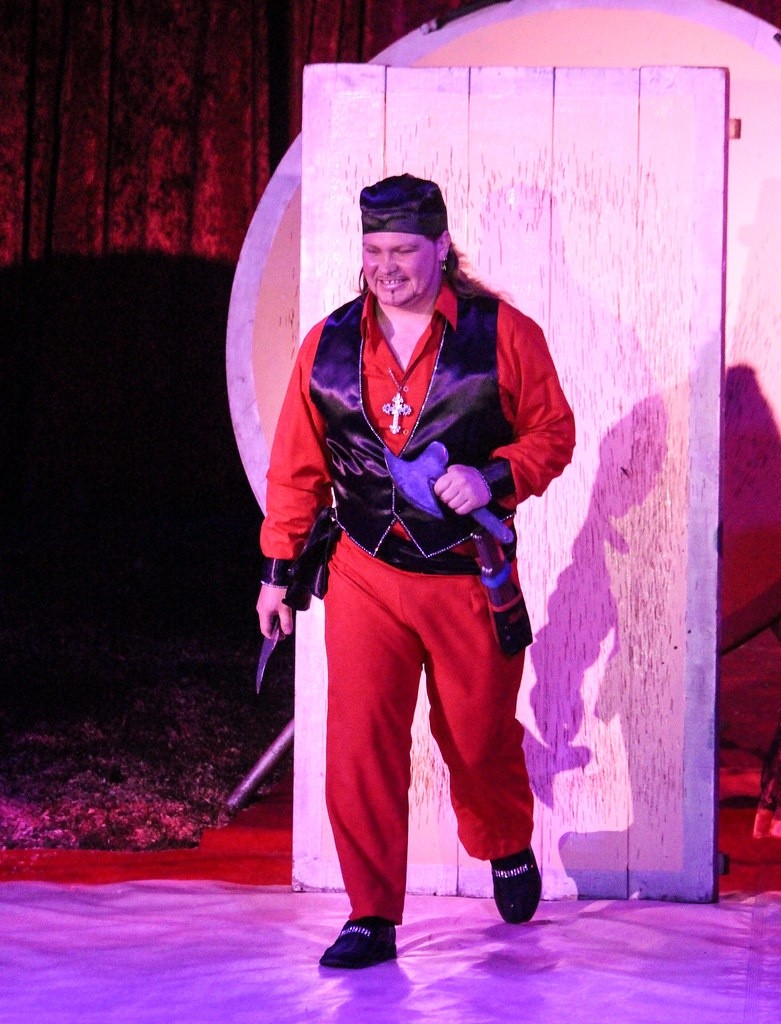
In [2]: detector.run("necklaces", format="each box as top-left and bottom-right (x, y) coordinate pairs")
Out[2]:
(381, 367), (412, 435)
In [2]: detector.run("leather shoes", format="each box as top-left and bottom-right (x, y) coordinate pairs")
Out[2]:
(319, 919), (397, 968)
(491, 844), (542, 925)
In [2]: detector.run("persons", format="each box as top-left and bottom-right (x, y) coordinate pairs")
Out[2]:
(255, 172), (578, 968)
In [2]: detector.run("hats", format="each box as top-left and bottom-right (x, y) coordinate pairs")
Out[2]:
(359, 173), (448, 236)
